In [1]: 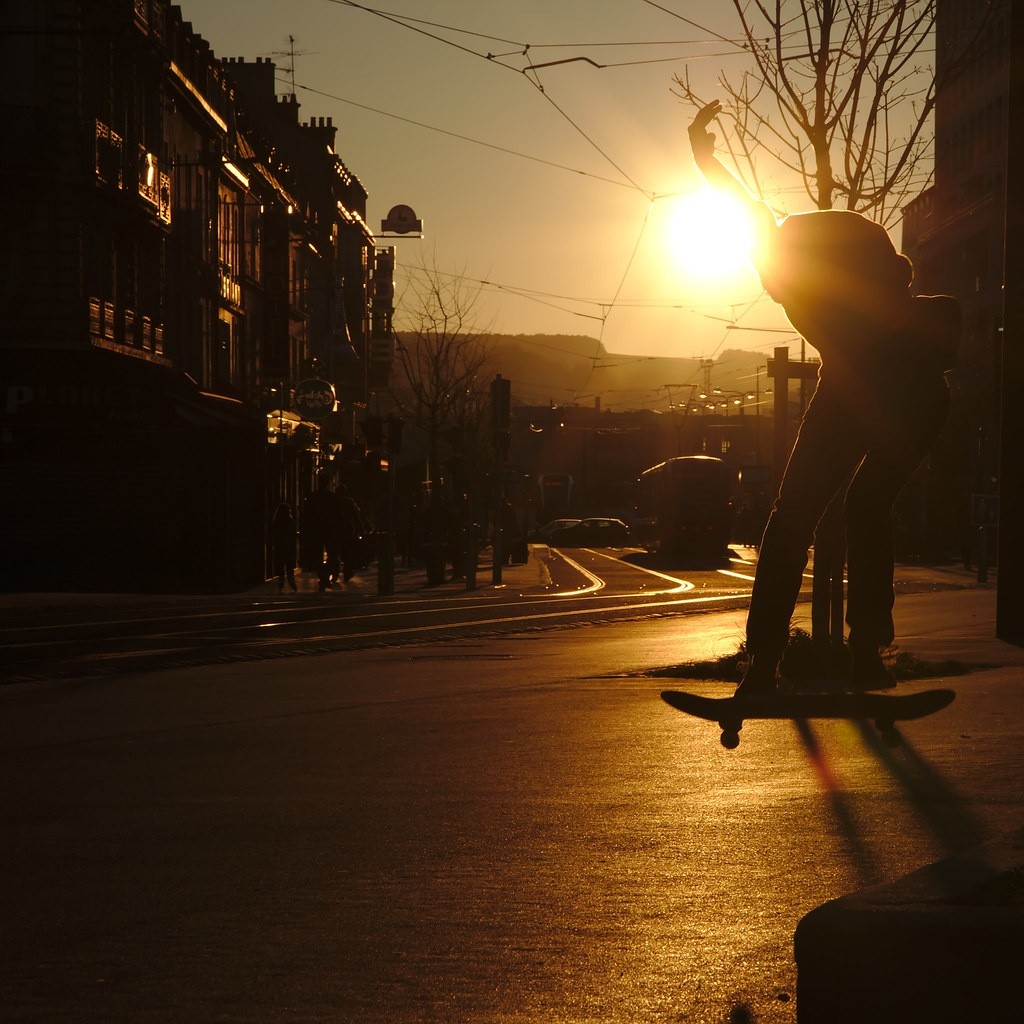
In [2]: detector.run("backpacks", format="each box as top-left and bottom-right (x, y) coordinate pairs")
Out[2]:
(779, 211), (913, 288)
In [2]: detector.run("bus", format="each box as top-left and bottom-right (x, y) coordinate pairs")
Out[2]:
(633, 456), (731, 558)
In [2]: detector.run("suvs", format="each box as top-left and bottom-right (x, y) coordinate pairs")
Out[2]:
(527, 519), (580, 538)
(549, 517), (631, 548)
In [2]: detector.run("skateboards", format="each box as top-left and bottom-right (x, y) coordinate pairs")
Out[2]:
(660, 687), (957, 750)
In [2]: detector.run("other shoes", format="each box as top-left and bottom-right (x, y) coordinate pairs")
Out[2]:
(734, 677), (794, 699)
(848, 647), (897, 691)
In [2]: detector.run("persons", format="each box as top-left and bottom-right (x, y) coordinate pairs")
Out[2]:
(731, 210), (968, 701)
(272, 474), (359, 592)
